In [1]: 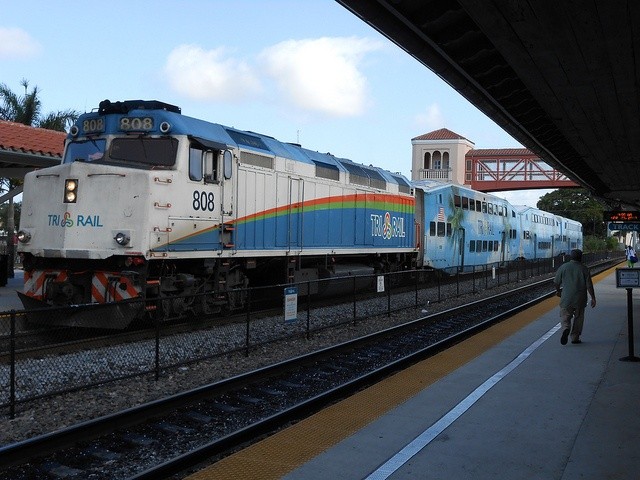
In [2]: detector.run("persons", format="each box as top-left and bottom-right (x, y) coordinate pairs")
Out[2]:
(627, 245), (638, 269)
(553, 249), (596, 346)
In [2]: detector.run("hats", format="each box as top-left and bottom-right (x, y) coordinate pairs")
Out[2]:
(571, 250), (583, 255)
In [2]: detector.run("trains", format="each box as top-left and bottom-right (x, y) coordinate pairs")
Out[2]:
(17, 99), (579, 327)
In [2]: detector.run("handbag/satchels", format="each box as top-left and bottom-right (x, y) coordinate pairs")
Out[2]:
(628, 251), (637, 262)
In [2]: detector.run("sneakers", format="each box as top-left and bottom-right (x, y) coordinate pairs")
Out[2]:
(560, 328), (569, 345)
(571, 339), (581, 344)
